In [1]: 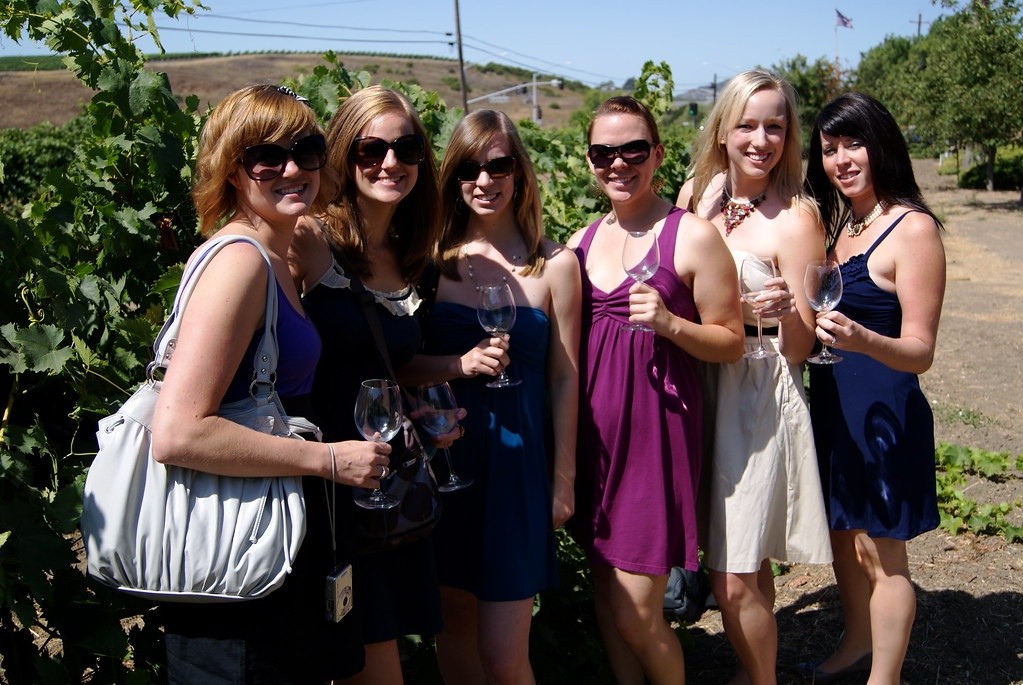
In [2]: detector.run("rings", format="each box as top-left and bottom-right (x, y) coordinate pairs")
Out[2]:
(456, 426), (464, 437)
(832, 337), (836, 346)
(379, 466), (385, 479)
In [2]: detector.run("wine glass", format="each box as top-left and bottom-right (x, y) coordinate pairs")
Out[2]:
(804, 260), (843, 364)
(739, 258), (780, 357)
(478, 283), (522, 388)
(354, 380), (403, 508)
(416, 379), (476, 494)
(622, 232), (661, 331)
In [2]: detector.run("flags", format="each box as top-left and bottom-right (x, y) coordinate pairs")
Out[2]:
(836, 9), (853, 28)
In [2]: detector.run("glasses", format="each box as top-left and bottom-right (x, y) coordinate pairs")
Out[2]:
(350, 134), (427, 168)
(455, 156), (518, 181)
(586, 139), (655, 169)
(236, 134), (328, 181)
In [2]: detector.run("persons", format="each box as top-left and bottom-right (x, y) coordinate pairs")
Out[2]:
(421, 109), (582, 685)
(150, 85), (392, 685)
(804, 92), (947, 685)
(567, 96), (746, 685)
(287, 85), (467, 685)
(677, 71), (834, 685)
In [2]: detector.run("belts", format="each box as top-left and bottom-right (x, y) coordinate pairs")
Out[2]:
(742, 323), (781, 338)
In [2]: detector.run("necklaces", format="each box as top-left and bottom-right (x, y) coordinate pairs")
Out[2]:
(464, 241), (520, 294)
(720, 180), (769, 237)
(848, 199), (888, 238)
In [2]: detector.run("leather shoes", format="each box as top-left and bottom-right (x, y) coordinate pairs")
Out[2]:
(791, 651), (872, 685)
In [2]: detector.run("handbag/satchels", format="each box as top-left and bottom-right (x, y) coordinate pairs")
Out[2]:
(662, 554), (713, 626)
(78, 235), (326, 606)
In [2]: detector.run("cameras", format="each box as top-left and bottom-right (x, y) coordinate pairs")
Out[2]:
(324, 562), (353, 623)
